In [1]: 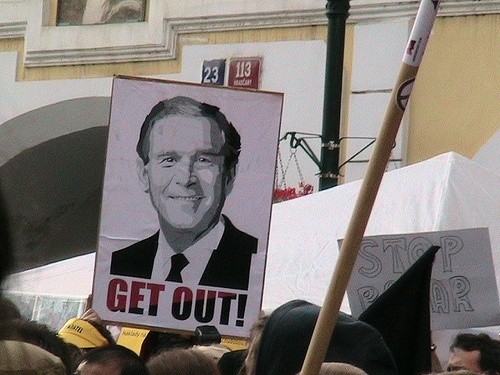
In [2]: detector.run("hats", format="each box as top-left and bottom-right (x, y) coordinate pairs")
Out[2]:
(58, 318), (109, 350)
(0, 339), (66, 374)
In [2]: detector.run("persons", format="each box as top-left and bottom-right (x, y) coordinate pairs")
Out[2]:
(0, 183), (500, 375)
